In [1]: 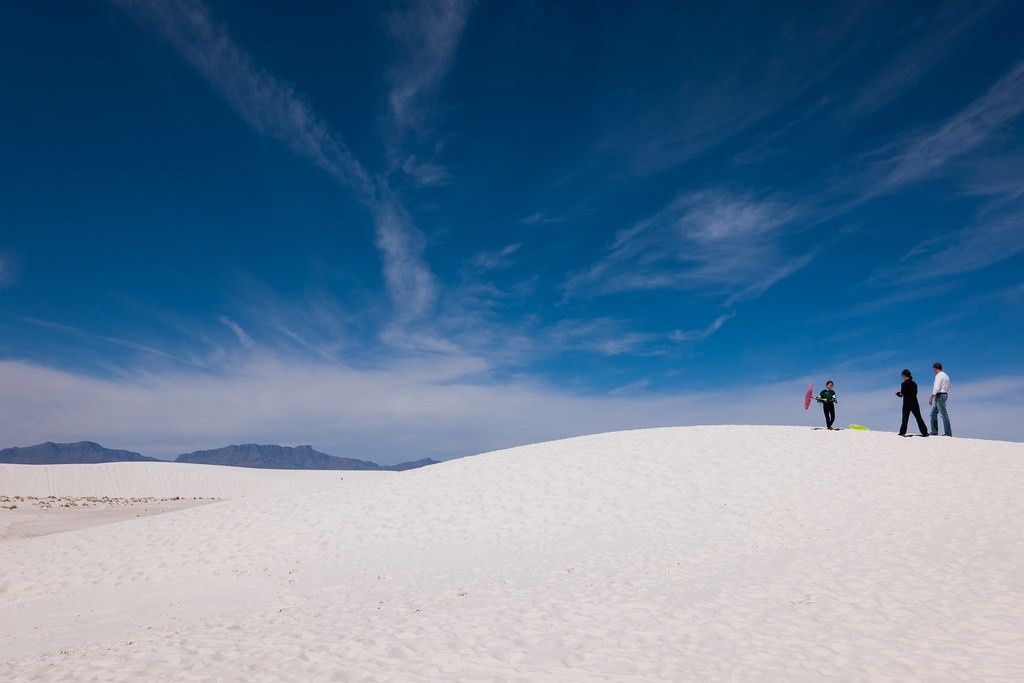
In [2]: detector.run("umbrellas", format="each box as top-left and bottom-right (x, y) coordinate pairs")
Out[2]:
(804, 384), (823, 409)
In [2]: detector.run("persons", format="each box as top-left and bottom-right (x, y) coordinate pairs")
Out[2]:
(896, 369), (929, 437)
(817, 380), (837, 430)
(928, 362), (952, 437)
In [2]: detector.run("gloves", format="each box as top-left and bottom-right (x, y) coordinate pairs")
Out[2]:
(896, 391), (902, 397)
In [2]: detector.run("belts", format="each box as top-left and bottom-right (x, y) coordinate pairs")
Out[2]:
(937, 393), (947, 394)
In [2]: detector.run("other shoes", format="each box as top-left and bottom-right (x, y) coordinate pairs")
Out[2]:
(921, 433), (929, 437)
(898, 433), (905, 436)
(929, 432), (938, 435)
(828, 426), (833, 430)
(942, 433), (952, 436)
